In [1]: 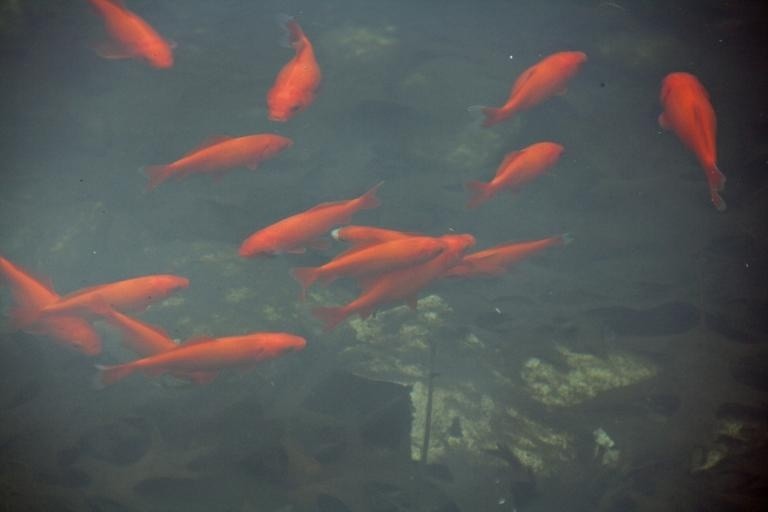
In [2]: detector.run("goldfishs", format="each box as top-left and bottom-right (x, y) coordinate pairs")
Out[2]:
(236, 179), (576, 333)
(0, 255), (309, 390)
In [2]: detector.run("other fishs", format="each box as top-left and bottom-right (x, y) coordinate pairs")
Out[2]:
(658, 71), (726, 212)
